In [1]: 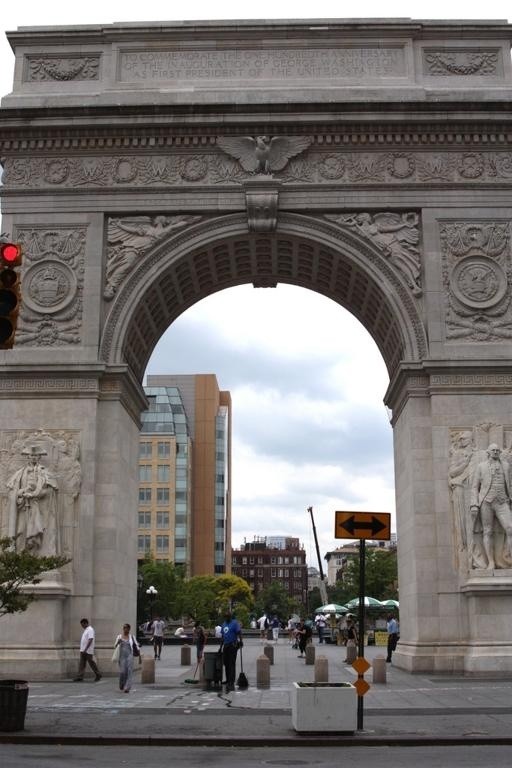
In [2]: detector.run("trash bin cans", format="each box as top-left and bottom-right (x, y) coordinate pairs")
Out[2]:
(0, 680), (29, 732)
(204, 652), (222, 689)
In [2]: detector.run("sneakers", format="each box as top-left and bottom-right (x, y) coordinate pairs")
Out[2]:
(94, 674), (102, 682)
(74, 678), (83, 681)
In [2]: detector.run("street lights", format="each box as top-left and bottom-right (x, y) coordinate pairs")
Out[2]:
(146, 586), (158, 619)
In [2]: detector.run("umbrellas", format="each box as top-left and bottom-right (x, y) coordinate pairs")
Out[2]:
(343, 596), (383, 617)
(378, 600), (399, 612)
(313, 604), (349, 614)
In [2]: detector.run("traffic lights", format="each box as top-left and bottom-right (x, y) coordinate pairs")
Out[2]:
(0, 242), (22, 349)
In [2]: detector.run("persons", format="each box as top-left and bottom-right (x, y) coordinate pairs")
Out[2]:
(192, 620), (206, 663)
(113, 624), (140, 692)
(0, 428), (80, 571)
(446, 430), (512, 570)
(138, 616), (167, 632)
(103, 215), (201, 300)
(214, 624), (223, 639)
(218, 610), (243, 694)
(71, 619), (102, 682)
(251, 612), (400, 665)
(174, 625), (185, 638)
(324, 213), (424, 299)
(151, 616), (165, 660)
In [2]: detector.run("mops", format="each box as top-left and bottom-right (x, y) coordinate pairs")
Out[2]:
(184, 654), (206, 685)
(236, 631), (250, 687)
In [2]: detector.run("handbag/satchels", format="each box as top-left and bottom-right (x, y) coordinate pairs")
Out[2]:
(112, 644), (120, 664)
(264, 617), (269, 629)
(133, 642), (140, 657)
